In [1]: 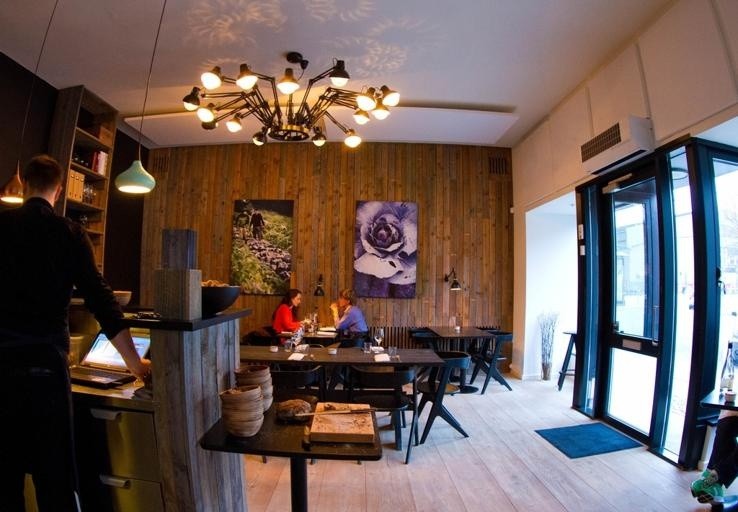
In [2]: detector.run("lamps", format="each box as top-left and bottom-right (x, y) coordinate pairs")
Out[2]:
(0, 0), (60, 206)
(313, 275), (326, 297)
(114, 2), (170, 197)
(444, 267), (464, 291)
(182, 50), (400, 150)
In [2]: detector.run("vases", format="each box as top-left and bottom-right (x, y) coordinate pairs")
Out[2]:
(542, 362), (552, 380)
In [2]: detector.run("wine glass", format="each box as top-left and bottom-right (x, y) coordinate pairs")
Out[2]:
(374, 328), (384, 348)
(292, 337), (302, 352)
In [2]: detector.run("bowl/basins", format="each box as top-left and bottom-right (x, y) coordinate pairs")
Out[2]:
(113, 290), (132, 305)
(201, 285), (240, 314)
(220, 363), (272, 438)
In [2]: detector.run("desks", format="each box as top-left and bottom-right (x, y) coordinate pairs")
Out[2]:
(198, 398), (382, 512)
(699, 386), (738, 412)
(556, 331), (576, 390)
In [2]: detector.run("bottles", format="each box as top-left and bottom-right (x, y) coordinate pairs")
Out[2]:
(718, 342), (735, 395)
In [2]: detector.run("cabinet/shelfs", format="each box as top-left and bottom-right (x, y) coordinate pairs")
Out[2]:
(46, 85), (120, 298)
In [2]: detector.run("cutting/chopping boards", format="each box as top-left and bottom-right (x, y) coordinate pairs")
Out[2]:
(310, 401), (374, 441)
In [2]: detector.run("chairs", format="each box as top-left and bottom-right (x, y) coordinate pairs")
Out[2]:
(244, 328), (512, 465)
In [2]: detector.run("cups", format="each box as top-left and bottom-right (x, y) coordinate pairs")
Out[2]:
(725, 392), (736, 402)
(388, 346), (397, 356)
(284, 341), (292, 352)
(364, 342), (372, 354)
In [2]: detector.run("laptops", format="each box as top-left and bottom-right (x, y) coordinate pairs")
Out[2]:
(67, 327), (153, 387)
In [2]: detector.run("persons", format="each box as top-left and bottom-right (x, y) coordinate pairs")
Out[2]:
(691, 409), (738, 503)
(0, 155), (153, 510)
(250, 208), (264, 241)
(324, 289), (369, 389)
(271, 289), (317, 394)
(237, 210), (250, 243)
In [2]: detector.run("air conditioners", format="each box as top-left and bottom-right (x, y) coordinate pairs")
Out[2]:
(579, 113), (655, 177)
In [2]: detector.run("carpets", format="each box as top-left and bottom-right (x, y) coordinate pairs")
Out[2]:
(533, 422), (644, 460)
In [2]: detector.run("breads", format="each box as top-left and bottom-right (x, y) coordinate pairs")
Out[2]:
(271, 399), (311, 423)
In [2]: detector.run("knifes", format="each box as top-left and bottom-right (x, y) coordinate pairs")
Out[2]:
(294, 408), (376, 416)
